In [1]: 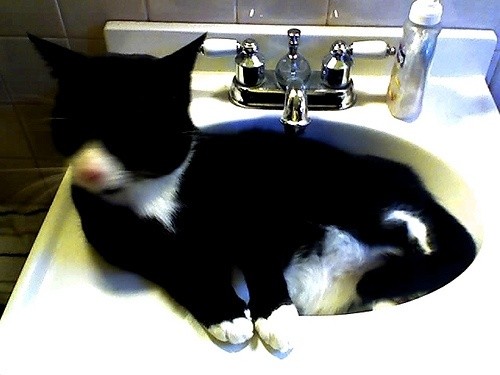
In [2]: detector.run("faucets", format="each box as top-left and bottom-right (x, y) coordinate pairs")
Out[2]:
(279, 23), (315, 130)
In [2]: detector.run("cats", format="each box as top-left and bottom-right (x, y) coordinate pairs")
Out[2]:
(26, 31), (478, 355)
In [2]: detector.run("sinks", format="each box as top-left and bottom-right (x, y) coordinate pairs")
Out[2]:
(0, 49), (500, 375)
